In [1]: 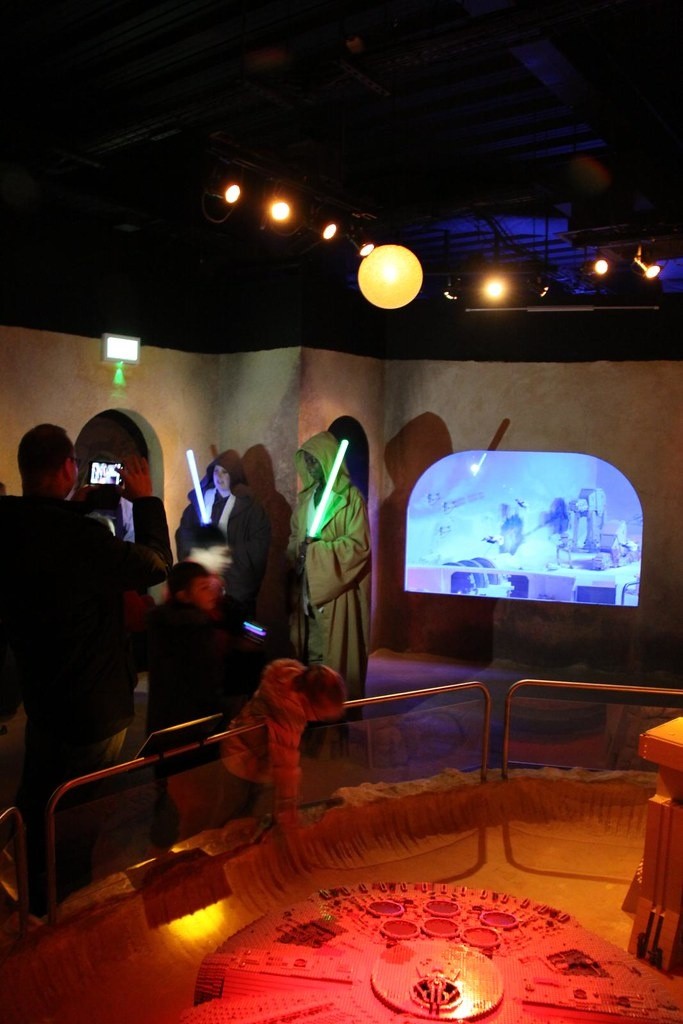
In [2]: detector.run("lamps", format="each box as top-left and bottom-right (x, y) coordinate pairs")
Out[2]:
(525, 278), (549, 298)
(356, 244), (423, 310)
(303, 203), (341, 244)
(443, 279), (464, 301)
(201, 172), (243, 224)
(631, 241), (661, 279)
(346, 225), (375, 258)
(101, 333), (141, 386)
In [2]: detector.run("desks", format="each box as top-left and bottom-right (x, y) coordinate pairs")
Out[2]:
(620, 716), (683, 974)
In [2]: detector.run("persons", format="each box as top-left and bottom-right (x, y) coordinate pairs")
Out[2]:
(284, 430), (372, 710)
(0, 424), (172, 918)
(176, 448), (275, 617)
(139, 559), (258, 857)
(212, 656), (348, 835)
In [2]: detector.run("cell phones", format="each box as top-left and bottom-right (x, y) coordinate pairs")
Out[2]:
(87, 461), (124, 487)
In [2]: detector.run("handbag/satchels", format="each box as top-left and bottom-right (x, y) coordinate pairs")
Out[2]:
(124, 590), (156, 632)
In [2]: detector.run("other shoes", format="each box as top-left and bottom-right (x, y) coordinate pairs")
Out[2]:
(145, 845), (173, 873)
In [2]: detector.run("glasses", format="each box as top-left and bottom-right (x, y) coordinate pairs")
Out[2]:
(61, 456), (81, 468)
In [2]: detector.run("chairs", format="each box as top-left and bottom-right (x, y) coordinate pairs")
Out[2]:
(442, 557), (515, 597)
